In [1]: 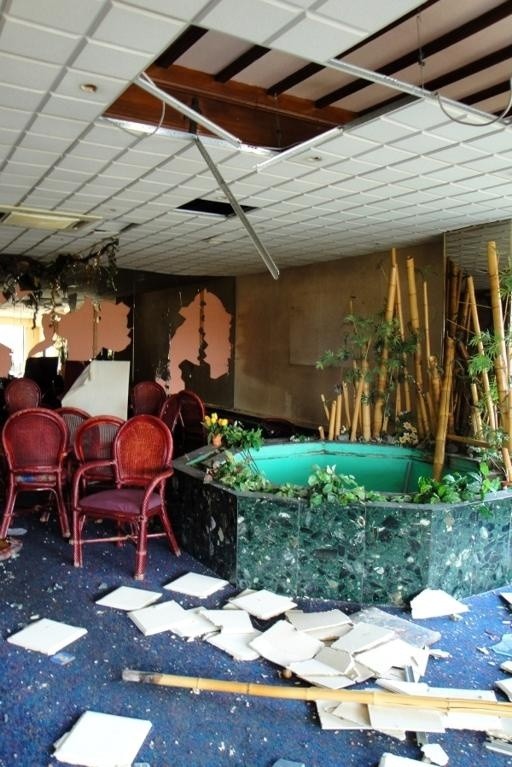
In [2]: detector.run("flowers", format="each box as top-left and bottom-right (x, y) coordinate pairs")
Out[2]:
(203, 412), (230, 444)
(51, 330), (68, 360)
(396, 420), (419, 447)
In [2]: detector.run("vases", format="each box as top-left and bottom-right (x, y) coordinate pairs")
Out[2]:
(56, 349), (64, 376)
(212, 434), (224, 448)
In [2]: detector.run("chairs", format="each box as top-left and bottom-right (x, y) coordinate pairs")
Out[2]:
(177, 387), (206, 443)
(2, 378), (42, 412)
(70, 415), (134, 550)
(158, 394), (183, 436)
(52, 404), (101, 484)
(2, 407), (71, 544)
(69, 412), (183, 582)
(129, 380), (167, 419)
